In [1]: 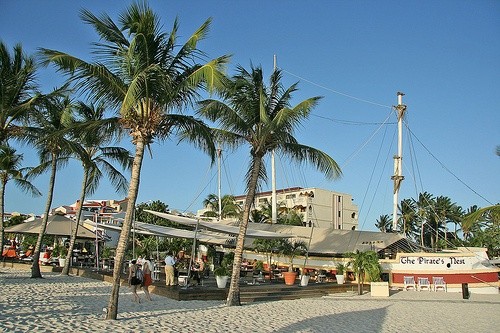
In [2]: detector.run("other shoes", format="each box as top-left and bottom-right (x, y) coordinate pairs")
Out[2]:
(145, 297), (152, 302)
(135, 297), (138, 301)
(138, 298), (141, 304)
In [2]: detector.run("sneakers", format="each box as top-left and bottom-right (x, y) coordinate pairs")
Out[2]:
(168, 284), (178, 286)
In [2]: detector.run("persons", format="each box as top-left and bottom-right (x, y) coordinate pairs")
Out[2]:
(165, 252), (176, 286)
(4, 238), (50, 265)
(141, 256), (153, 302)
(188, 258), (205, 281)
(128, 259), (142, 304)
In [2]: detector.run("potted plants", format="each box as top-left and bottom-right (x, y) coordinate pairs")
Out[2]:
(58, 246), (68, 267)
(280, 239), (307, 286)
(333, 259), (351, 284)
(214, 267), (232, 288)
(222, 252), (235, 268)
(51, 246), (58, 262)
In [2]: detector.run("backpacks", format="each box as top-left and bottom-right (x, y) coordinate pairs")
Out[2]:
(135, 267), (144, 284)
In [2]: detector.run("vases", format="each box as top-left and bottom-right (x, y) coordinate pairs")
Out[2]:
(299, 275), (310, 286)
(253, 261), (263, 274)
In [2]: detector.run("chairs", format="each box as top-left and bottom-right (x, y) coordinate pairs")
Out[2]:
(403, 276), (417, 292)
(433, 277), (447, 293)
(418, 277), (431, 292)
